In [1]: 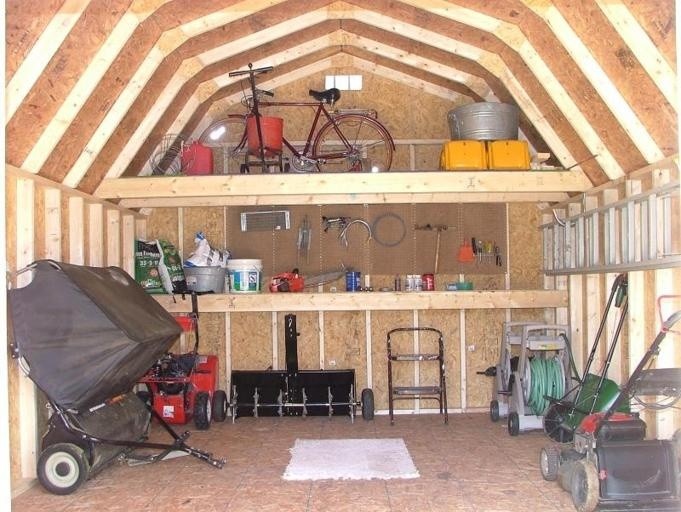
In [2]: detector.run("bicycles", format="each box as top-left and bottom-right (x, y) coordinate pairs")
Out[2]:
(196, 62), (398, 175)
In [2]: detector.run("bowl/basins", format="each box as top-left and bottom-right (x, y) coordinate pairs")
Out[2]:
(447, 101), (520, 141)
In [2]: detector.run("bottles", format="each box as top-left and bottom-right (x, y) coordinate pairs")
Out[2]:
(415, 275), (422, 292)
(478, 240), (482, 254)
(395, 273), (401, 291)
(404, 274), (413, 291)
(412, 274), (416, 291)
(483, 241), (492, 253)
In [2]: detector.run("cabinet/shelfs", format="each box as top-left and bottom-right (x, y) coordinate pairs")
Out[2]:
(95, 170), (591, 310)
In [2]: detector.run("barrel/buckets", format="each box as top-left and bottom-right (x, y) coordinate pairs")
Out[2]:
(227, 259), (263, 293)
(181, 141), (214, 176)
(248, 116), (284, 157)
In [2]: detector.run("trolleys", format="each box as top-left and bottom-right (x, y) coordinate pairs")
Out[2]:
(136, 286), (228, 431)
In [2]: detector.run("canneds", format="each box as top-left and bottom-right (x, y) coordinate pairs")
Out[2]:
(346, 271), (361, 292)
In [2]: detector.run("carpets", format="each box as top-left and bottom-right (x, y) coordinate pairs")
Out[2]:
(281, 438), (421, 481)
